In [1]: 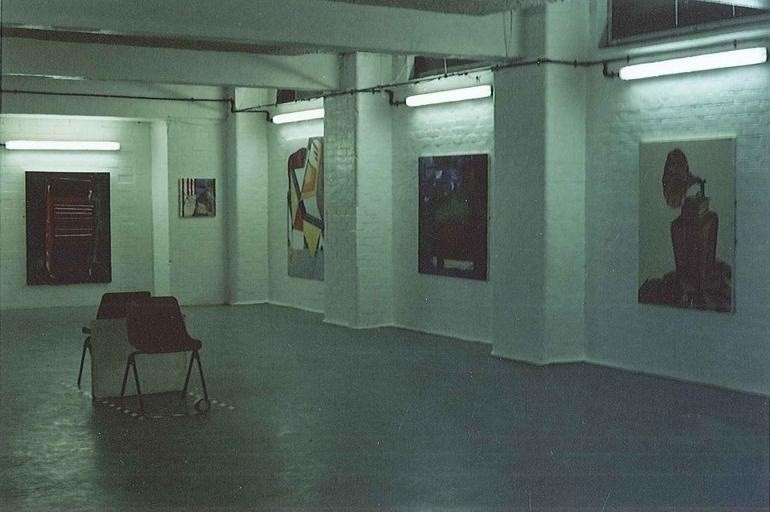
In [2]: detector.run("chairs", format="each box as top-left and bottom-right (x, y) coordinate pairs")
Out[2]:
(76, 287), (214, 420)
